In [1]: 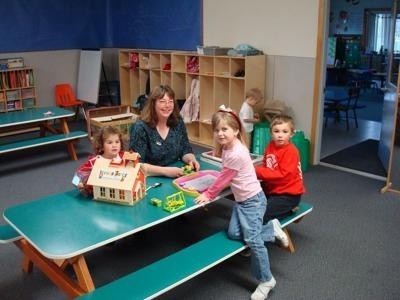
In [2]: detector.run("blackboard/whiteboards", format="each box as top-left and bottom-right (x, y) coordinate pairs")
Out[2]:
(76, 48), (103, 106)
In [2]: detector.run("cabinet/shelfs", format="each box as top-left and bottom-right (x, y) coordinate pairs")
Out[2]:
(118, 45), (266, 148)
(0, 66), (39, 112)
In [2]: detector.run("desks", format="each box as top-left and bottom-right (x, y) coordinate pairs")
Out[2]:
(2, 153), (267, 299)
(0, 106), (76, 133)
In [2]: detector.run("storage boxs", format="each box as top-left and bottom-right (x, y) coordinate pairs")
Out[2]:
(203, 46), (234, 55)
(196, 46), (203, 54)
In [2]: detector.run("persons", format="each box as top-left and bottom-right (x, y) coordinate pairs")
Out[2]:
(193, 104), (290, 300)
(239, 88), (263, 151)
(78, 125), (125, 195)
(240, 114), (306, 258)
(128, 84), (201, 178)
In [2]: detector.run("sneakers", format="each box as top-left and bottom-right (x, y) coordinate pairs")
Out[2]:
(272, 218), (289, 248)
(251, 277), (276, 300)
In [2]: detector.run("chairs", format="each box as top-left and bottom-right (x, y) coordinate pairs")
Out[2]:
(54, 83), (83, 107)
(325, 88), (361, 131)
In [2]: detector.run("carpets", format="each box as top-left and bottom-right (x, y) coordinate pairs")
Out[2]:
(319, 137), (390, 180)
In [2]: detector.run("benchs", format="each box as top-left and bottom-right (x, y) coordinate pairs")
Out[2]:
(0, 225), (24, 244)
(0, 130), (89, 162)
(73, 198), (313, 300)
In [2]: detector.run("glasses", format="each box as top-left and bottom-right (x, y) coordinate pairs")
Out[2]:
(155, 99), (174, 105)
